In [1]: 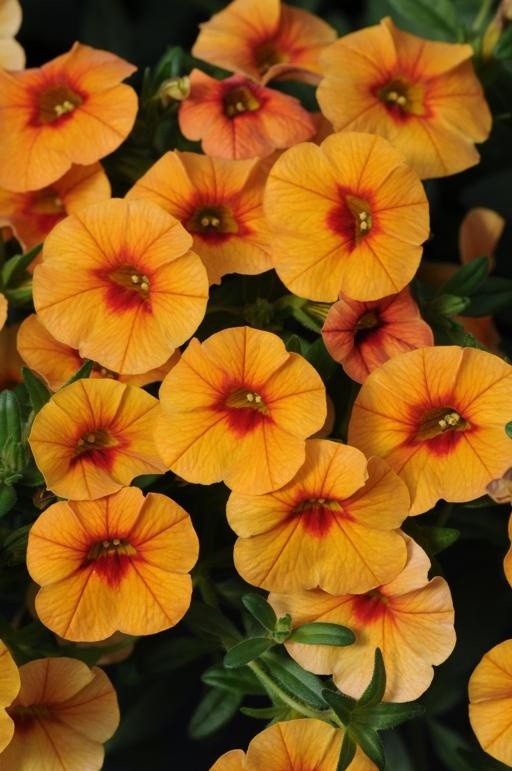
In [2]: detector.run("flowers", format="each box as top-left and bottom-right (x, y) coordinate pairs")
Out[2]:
(1, 0), (512, 771)
